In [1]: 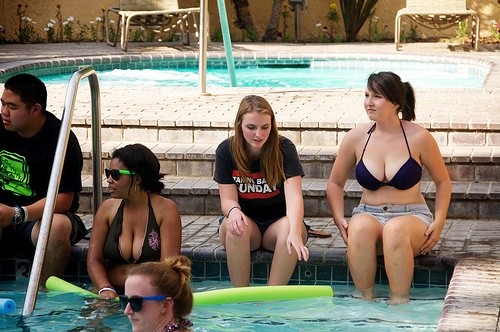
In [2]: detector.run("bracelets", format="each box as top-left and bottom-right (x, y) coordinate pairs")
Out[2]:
(99, 285), (116, 295)
(227, 207), (240, 218)
(19, 206), (28, 223)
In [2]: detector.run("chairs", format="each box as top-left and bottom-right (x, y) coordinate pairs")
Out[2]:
(394, 0), (480, 52)
(104, 0), (200, 52)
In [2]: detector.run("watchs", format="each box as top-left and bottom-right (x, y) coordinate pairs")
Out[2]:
(12, 207), (22, 226)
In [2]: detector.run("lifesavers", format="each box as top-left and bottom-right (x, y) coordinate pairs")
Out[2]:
(45, 275), (333, 305)
(0, 297), (16, 315)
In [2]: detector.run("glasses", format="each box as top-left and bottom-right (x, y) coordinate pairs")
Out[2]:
(119, 295), (167, 312)
(105, 169), (136, 181)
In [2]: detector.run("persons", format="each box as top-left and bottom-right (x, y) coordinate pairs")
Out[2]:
(213, 95), (310, 287)
(0, 73), (90, 286)
(124, 256), (194, 332)
(87, 144), (182, 298)
(327, 71), (452, 292)
